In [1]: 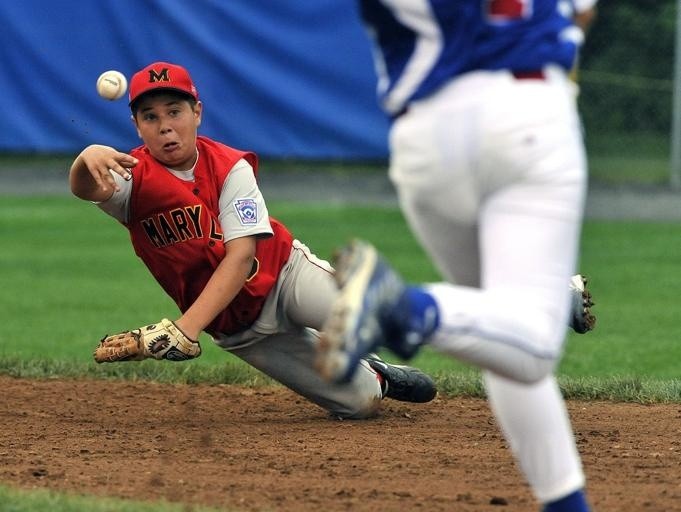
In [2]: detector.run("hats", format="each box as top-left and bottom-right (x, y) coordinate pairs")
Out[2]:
(128, 60), (199, 107)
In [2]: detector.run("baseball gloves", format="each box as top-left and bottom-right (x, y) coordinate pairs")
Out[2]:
(94, 318), (201, 365)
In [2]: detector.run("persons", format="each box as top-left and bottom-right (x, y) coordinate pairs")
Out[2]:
(68, 61), (597, 426)
(311, 0), (607, 511)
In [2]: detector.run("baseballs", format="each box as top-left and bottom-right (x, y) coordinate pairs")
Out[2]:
(97, 71), (128, 101)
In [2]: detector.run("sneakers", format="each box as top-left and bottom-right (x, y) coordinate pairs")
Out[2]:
(568, 273), (594, 335)
(315, 234), (439, 386)
(358, 346), (438, 403)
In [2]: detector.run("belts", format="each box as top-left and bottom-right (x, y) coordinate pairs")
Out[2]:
(394, 65), (545, 119)
